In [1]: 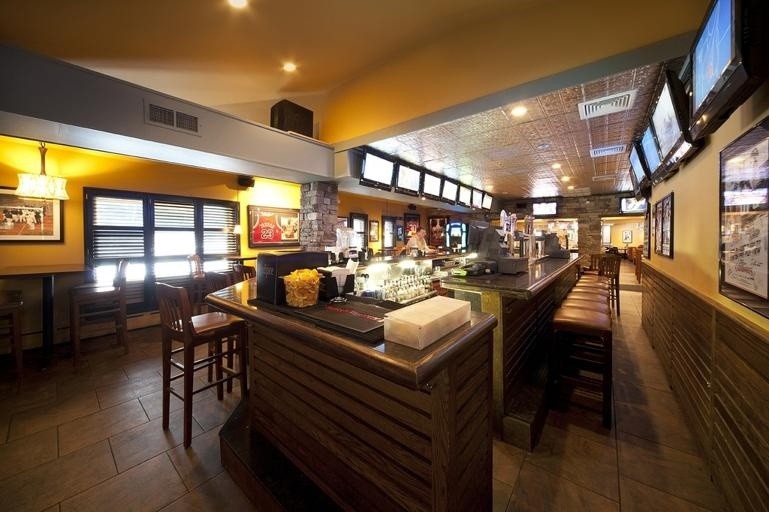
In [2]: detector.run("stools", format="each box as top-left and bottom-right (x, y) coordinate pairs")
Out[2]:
(537, 274), (612, 442)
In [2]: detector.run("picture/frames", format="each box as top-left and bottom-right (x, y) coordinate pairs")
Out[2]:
(654, 199), (662, 255)
(369, 221), (379, 241)
(428, 216), (450, 248)
(403, 212), (421, 245)
(662, 192), (673, 260)
(247, 205), (300, 248)
(718, 114), (769, 321)
(337, 216), (349, 228)
(0, 186), (64, 244)
(622, 231), (632, 243)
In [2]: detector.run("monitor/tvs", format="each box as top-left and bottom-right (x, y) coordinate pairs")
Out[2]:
(625, 0), (759, 202)
(361, 150), (496, 210)
(532, 202), (557, 217)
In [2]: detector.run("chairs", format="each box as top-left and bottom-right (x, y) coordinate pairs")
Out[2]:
(582, 244), (644, 318)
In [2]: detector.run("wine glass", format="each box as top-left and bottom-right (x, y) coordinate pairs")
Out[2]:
(382, 270), (435, 303)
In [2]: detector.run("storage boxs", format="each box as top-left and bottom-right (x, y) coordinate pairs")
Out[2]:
(383, 295), (472, 351)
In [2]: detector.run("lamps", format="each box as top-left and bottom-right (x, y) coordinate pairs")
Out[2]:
(14, 140), (70, 201)
(231, 189), (243, 237)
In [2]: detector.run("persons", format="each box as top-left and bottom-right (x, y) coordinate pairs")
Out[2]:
(404, 226), (438, 258)
